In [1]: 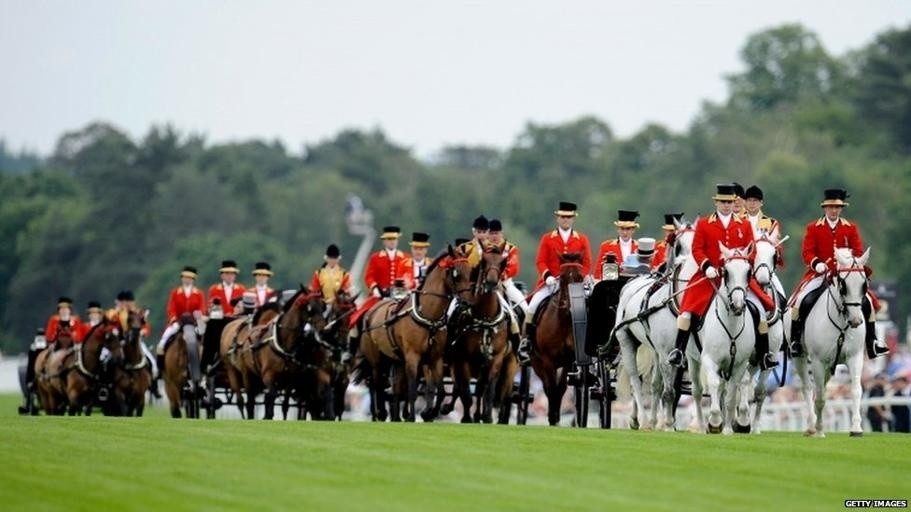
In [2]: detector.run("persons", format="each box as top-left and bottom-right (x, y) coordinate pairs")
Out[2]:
(24, 183), (911, 432)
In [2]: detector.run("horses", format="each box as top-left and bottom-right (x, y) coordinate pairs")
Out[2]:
(47, 313), (126, 417)
(613, 213), (701, 431)
(722, 230), (788, 430)
(219, 282), (326, 416)
(474, 304), (521, 424)
(448, 238), (508, 424)
(162, 312), (206, 417)
(353, 242), (476, 422)
(33, 319), (75, 414)
(783, 245), (872, 438)
(530, 245), (585, 427)
(99, 305), (153, 417)
(282, 288), (362, 421)
(380, 312), (459, 423)
(685, 239), (760, 434)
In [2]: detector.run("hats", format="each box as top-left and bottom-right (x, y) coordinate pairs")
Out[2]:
(554, 201), (577, 216)
(821, 189), (850, 206)
(614, 209), (640, 228)
(634, 237), (657, 258)
(743, 185), (763, 200)
(326, 244), (340, 258)
(125, 290), (134, 299)
(488, 219), (502, 232)
(58, 297), (73, 308)
(218, 260), (239, 273)
(662, 213), (685, 231)
(408, 232), (431, 247)
(733, 183), (744, 198)
(472, 215), (489, 230)
(252, 262), (273, 277)
(180, 266), (198, 279)
(117, 292), (125, 300)
(87, 301), (102, 312)
(380, 226), (400, 238)
(712, 184), (736, 201)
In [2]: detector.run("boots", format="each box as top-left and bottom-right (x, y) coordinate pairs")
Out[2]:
(786, 319), (804, 359)
(866, 321), (890, 359)
(755, 332), (780, 372)
(511, 333), (521, 356)
(343, 337), (357, 363)
(148, 379), (161, 399)
(156, 353), (166, 380)
(516, 322), (536, 361)
(667, 328), (691, 365)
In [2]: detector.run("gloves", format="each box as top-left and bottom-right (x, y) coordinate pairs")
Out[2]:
(545, 275), (555, 285)
(815, 262), (829, 274)
(705, 266), (717, 279)
(372, 287), (381, 299)
